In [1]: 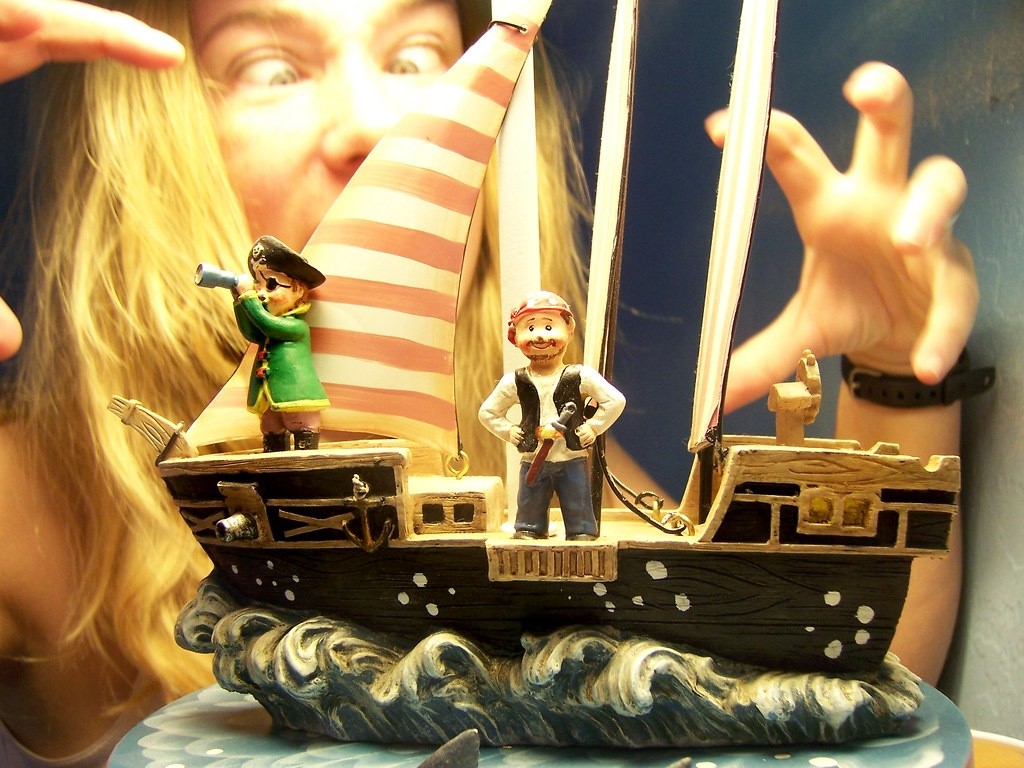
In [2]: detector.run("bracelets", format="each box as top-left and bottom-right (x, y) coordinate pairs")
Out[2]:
(841, 346), (996, 407)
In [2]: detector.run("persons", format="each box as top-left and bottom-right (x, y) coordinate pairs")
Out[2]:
(478, 291), (626, 541)
(0, 0), (996, 768)
(196, 234), (332, 453)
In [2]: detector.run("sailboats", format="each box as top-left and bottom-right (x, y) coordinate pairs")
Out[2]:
(105, 0), (963, 681)
